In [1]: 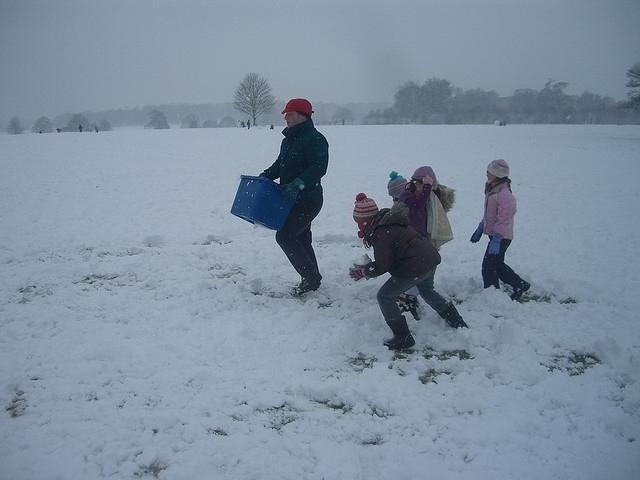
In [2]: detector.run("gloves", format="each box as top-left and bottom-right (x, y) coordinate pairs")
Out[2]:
(276, 177), (305, 202)
(348, 264), (366, 281)
(470, 223), (483, 243)
(488, 233), (504, 255)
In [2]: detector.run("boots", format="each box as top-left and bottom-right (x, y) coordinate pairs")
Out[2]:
(506, 274), (530, 300)
(383, 315), (415, 348)
(440, 303), (468, 328)
(395, 292), (422, 321)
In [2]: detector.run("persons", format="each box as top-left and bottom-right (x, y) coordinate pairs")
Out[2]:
(78, 123), (83, 132)
(350, 192), (467, 351)
(247, 119), (251, 130)
(388, 171), (408, 199)
(260, 100), (330, 295)
(470, 160), (530, 300)
(399, 167), (448, 320)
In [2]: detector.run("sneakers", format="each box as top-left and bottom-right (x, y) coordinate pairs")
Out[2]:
(290, 279), (312, 297)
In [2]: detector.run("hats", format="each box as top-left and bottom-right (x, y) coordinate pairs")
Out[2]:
(353, 193), (379, 238)
(387, 170), (407, 204)
(281, 99), (314, 117)
(487, 159), (509, 179)
(411, 166), (437, 186)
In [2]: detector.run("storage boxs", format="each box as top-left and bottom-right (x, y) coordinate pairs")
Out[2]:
(229, 174), (297, 232)
